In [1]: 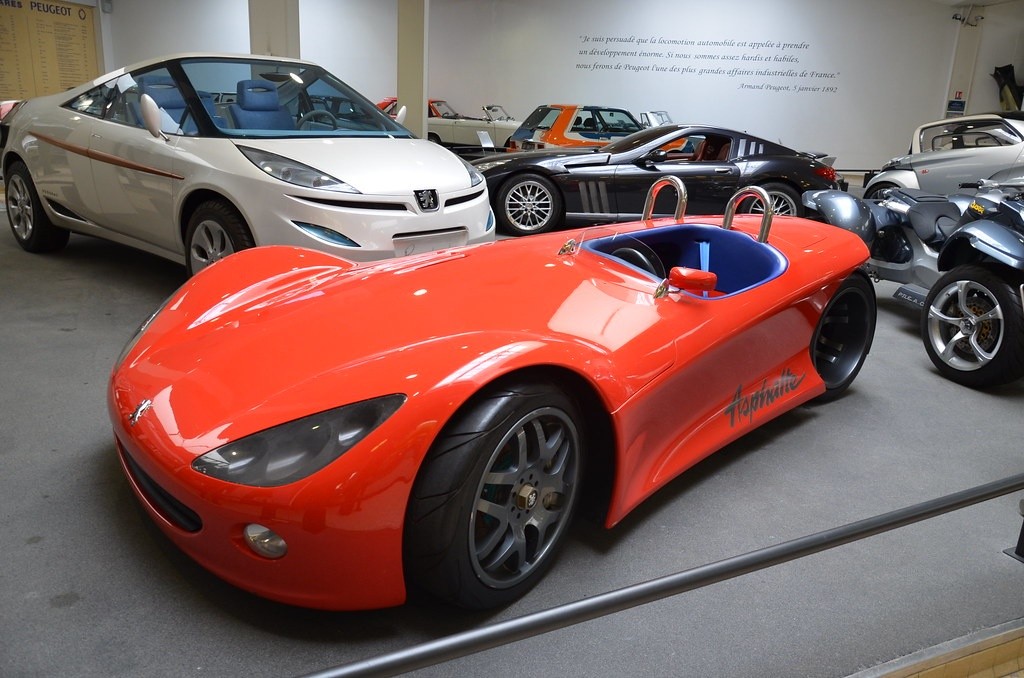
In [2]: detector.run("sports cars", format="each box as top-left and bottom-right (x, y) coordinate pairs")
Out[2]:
(473, 123), (843, 236)
(864, 129), (1023, 199)
(427, 104), (524, 152)
(101, 176), (878, 625)
(1, 52), (496, 277)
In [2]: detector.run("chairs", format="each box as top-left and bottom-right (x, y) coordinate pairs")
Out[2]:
(571, 116), (583, 131)
(688, 140), (707, 160)
(129, 74), (198, 135)
(717, 143), (731, 161)
(227, 79), (297, 130)
(197, 91), (227, 131)
(583, 118), (597, 131)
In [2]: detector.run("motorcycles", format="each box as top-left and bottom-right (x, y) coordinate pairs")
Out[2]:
(799, 187), (1023, 383)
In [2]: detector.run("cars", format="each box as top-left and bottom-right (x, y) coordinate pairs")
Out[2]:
(506, 104), (694, 155)
(376, 97), (459, 123)
